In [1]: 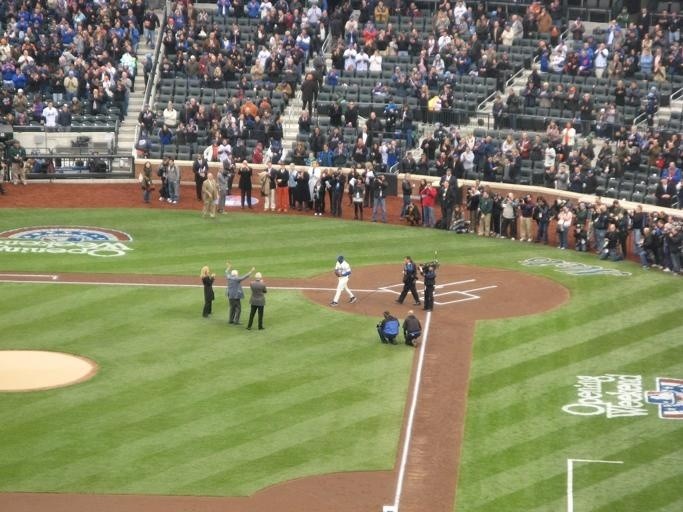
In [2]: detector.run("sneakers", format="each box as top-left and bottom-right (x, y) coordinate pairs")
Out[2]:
(391, 337), (418, 347)
(159, 196), (178, 205)
(314, 212), (323, 216)
(651, 263), (674, 274)
(500, 234), (535, 243)
(348, 297), (357, 303)
(330, 302), (338, 307)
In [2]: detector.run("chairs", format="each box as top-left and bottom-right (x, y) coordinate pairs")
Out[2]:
(135, 3), (682, 205)
(3, 1), (143, 133)
